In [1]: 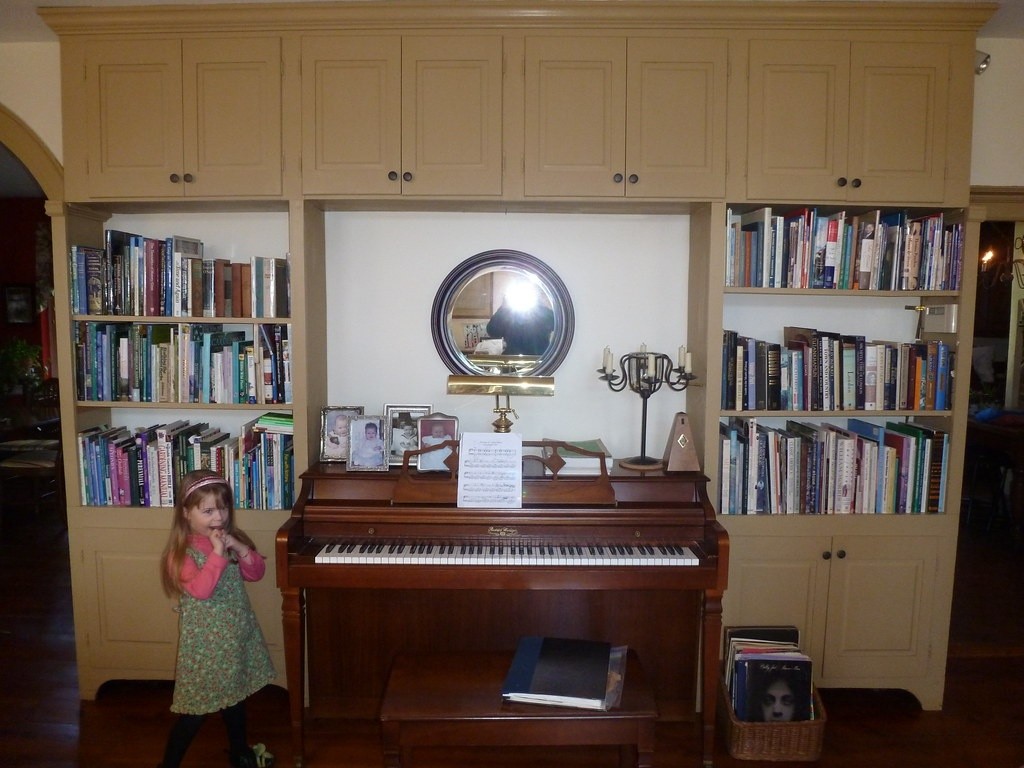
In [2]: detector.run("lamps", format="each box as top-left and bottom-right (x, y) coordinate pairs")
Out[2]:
(443, 370), (555, 432)
(467, 354), (542, 373)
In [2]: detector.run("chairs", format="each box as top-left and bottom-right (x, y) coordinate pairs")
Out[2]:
(2, 423), (68, 544)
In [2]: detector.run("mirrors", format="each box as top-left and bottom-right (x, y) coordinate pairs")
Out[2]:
(433, 250), (571, 381)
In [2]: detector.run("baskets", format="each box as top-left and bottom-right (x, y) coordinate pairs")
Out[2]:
(718, 658), (826, 761)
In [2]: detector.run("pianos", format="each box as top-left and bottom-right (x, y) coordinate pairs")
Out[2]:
(275, 458), (730, 768)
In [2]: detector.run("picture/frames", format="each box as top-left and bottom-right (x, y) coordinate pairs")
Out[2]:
(5, 284), (38, 323)
(319, 404), (365, 462)
(416, 413), (460, 472)
(346, 415), (390, 472)
(382, 403), (433, 465)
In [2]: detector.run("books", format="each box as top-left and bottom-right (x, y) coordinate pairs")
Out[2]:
(725, 625), (813, 723)
(502, 634), (626, 711)
(717, 417), (950, 513)
(72, 320), (292, 404)
(725, 206), (964, 291)
(722, 327), (956, 412)
(77, 411), (295, 511)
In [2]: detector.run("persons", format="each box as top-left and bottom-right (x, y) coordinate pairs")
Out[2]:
(157, 469), (276, 768)
(393, 422), (418, 457)
(354, 422), (384, 466)
(421, 423), (452, 470)
(69, 229), (292, 318)
(487, 296), (555, 356)
(327, 415), (347, 456)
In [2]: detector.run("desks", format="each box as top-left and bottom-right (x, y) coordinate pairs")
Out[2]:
(962, 411), (1024, 531)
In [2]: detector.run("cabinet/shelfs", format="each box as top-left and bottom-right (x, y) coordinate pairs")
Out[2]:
(300, 23), (506, 197)
(683, 205), (987, 710)
(56, 198), (323, 700)
(739, 33), (950, 200)
(522, 31), (728, 200)
(81, 27), (279, 196)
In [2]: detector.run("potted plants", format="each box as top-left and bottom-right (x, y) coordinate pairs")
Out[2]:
(3, 337), (45, 393)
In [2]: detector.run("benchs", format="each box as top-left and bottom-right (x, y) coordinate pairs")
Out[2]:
(379, 652), (659, 768)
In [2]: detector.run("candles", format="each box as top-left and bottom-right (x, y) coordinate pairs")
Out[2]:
(646, 354), (656, 377)
(603, 345), (612, 366)
(630, 354), (639, 380)
(642, 342), (647, 353)
(608, 355), (614, 372)
(678, 344), (685, 368)
(685, 352), (692, 373)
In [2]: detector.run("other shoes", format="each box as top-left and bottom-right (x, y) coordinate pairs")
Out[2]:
(230, 744), (273, 768)
